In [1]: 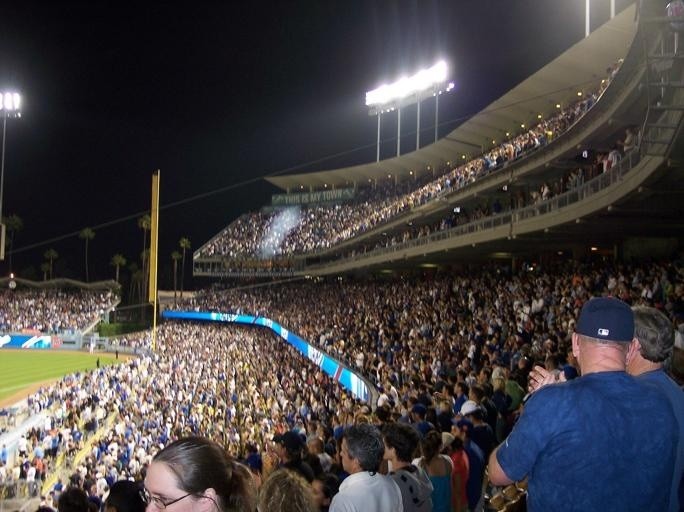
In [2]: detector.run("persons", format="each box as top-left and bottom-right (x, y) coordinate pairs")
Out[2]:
(665, 0), (684, 31)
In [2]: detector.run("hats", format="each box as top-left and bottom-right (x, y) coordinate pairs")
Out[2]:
(248, 454), (263, 470)
(554, 329), (567, 340)
(400, 405), (436, 434)
(458, 399), (481, 415)
(559, 365), (578, 381)
(575, 295), (635, 344)
(274, 431), (305, 450)
(329, 425), (354, 454)
(438, 418), (474, 452)
(326, 326), (515, 394)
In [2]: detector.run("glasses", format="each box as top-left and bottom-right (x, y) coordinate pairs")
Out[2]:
(138, 491), (194, 508)
